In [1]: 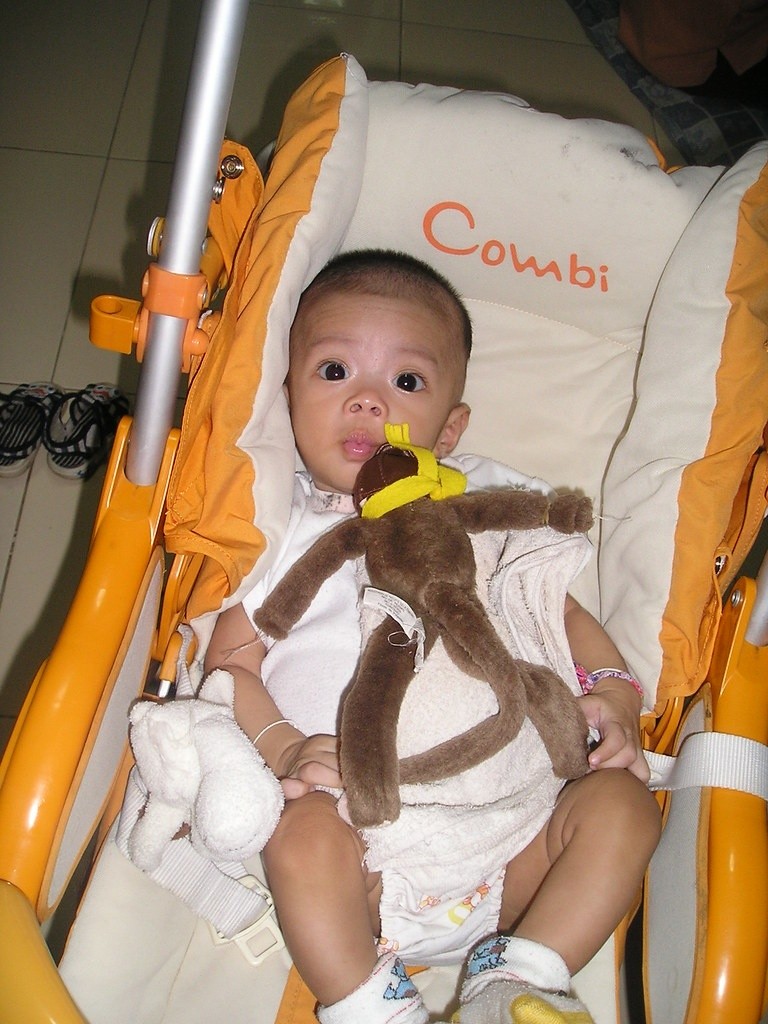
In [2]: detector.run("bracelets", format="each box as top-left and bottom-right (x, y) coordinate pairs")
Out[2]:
(574, 662), (644, 709)
(252, 719), (296, 746)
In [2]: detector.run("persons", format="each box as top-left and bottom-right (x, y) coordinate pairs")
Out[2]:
(205, 248), (662, 1024)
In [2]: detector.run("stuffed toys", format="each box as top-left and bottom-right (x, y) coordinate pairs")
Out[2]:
(129, 668), (285, 869)
(252, 447), (593, 831)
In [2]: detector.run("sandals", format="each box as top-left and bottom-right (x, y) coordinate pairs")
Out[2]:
(42, 381), (131, 483)
(0, 382), (67, 478)
